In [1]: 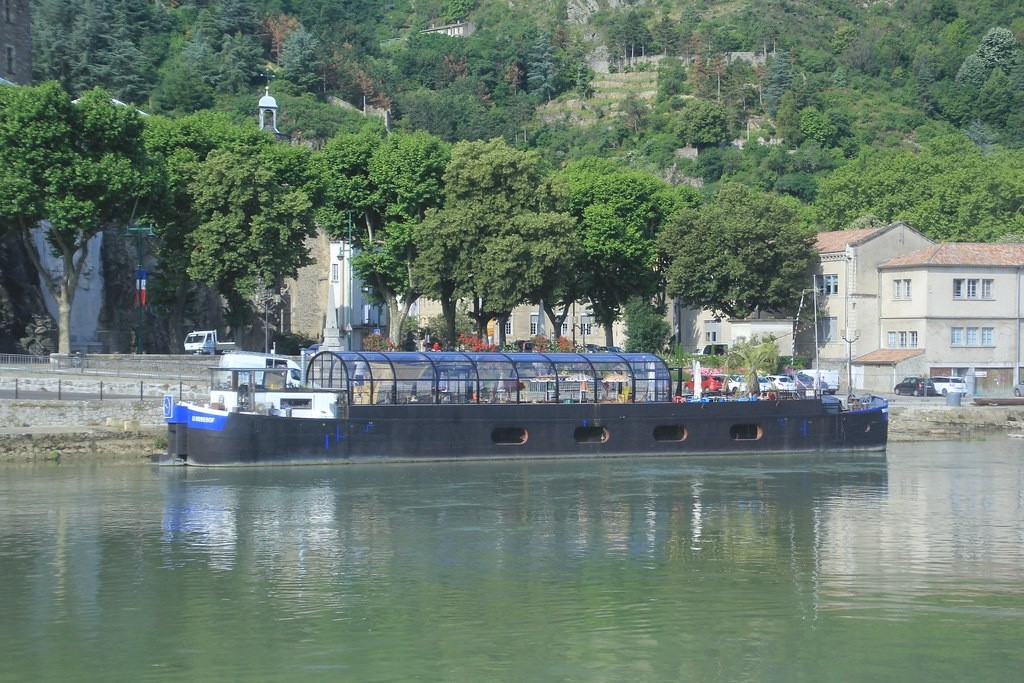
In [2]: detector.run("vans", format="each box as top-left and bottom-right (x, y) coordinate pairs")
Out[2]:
(797, 368), (839, 395)
(218, 349), (324, 388)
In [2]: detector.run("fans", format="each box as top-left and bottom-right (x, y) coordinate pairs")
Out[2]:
(458, 333), (496, 352)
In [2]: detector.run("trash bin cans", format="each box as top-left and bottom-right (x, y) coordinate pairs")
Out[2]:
(947, 392), (962, 405)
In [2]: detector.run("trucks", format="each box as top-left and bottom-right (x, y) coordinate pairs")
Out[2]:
(184, 329), (237, 356)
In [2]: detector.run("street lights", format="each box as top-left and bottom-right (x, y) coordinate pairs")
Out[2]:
(336, 212), (353, 351)
(840, 329), (862, 394)
(123, 222), (157, 355)
(256, 294), (281, 359)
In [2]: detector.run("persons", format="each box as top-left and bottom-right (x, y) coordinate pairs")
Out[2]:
(434, 342), (440, 350)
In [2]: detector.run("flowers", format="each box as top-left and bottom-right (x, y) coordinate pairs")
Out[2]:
(550, 336), (577, 352)
(529, 334), (552, 353)
(363, 334), (399, 351)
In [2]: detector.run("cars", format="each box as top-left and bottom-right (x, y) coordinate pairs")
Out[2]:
(718, 373), (827, 396)
(1012, 381), (1024, 397)
(930, 375), (967, 398)
(893, 376), (936, 396)
(684, 374), (728, 392)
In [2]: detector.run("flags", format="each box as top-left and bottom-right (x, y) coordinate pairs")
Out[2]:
(134, 269), (140, 306)
(139, 271), (147, 305)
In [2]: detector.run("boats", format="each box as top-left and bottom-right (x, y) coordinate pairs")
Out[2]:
(162, 274), (891, 468)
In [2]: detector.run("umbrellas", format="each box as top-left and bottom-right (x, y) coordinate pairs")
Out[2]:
(438, 371), (447, 402)
(497, 373), (505, 402)
(566, 372), (629, 403)
(538, 299), (547, 336)
(530, 373), (561, 402)
